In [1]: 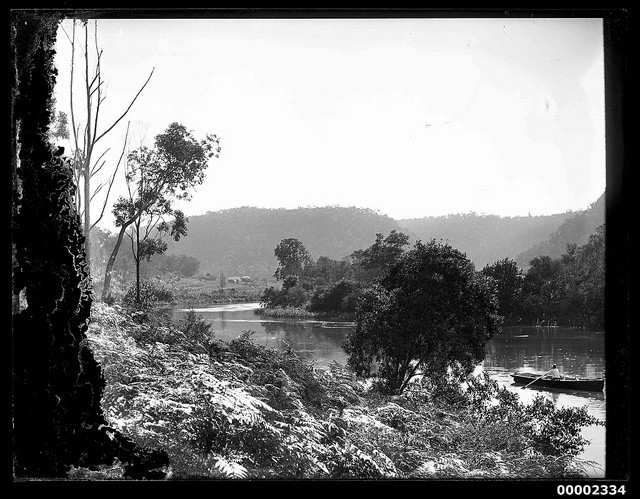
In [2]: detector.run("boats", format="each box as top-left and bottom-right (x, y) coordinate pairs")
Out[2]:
(509, 370), (605, 394)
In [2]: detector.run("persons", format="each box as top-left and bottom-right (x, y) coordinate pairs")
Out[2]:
(544, 364), (560, 380)
